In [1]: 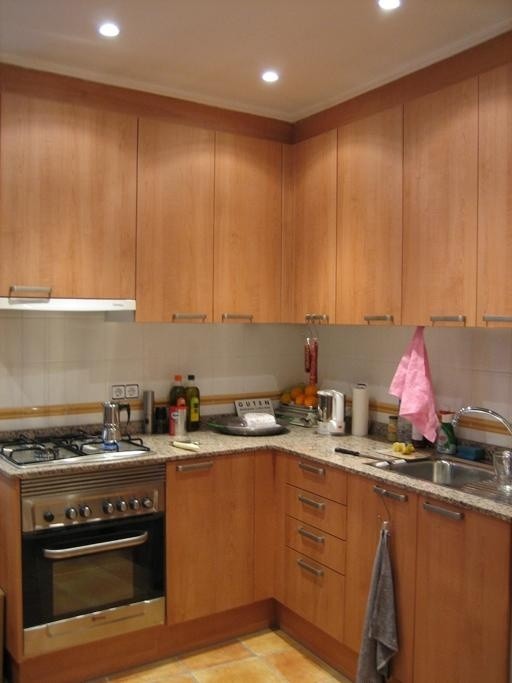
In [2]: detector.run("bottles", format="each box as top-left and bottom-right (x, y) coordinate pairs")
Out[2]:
(154, 407), (168, 434)
(386, 416), (397, 442)
(143, 390), (154, 433)
(183, 374), (201, 431)
(168, 375), (187, 435)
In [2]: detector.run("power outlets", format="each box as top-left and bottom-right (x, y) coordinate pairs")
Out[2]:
(127, 386), (140, 400)
(113, 386), (130, 400)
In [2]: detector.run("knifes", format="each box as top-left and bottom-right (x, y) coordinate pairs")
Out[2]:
(335, 447), (397, 463)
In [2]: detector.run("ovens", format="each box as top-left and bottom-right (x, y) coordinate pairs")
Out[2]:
(19, 462), (167, 659)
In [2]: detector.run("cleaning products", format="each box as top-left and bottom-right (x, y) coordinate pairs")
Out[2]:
(436, 410), (457, 455)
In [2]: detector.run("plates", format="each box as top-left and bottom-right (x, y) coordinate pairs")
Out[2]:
(207, 417), (284, 436)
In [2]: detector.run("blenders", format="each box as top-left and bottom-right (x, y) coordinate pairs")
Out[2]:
(314, 389), (345, 435)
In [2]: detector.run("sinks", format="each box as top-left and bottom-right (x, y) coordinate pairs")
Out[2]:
(361, 453), (497, 490)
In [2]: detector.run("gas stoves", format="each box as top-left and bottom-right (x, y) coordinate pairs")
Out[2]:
(0, 428), (149, 465)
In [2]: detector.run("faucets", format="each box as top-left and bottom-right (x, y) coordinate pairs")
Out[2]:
(450, 405), (512, 436)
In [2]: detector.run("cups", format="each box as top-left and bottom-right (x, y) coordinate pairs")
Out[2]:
(492, 451), (512, 496)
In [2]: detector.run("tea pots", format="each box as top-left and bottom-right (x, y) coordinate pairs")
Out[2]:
(101, 398), (131, 442)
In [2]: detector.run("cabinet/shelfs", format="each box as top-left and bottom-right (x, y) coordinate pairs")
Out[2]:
(275, 450), (346, 673)
(164, 453), (255, 655)
(289, 78), (404, 327)
(405, 32), (512, 328)
(137, 89), (283, 323)
(348, 474), (512, 683)
(1, 63), (141, 297)
